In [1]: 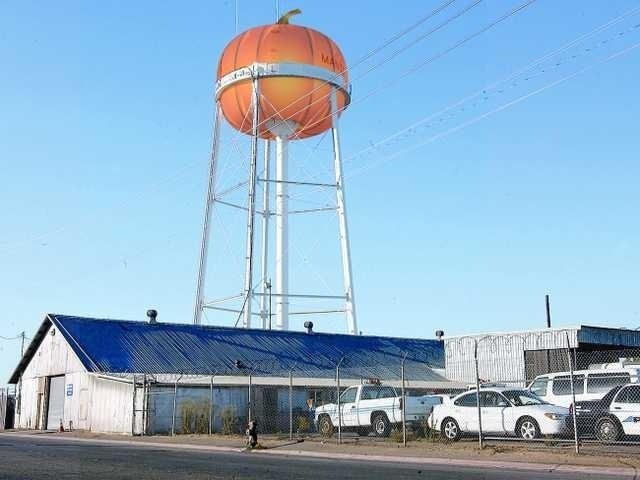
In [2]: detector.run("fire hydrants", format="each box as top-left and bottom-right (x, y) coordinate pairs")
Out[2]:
(246, 420), (259, 448)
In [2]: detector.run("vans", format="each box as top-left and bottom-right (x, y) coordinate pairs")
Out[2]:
(522, 367), (640, 409)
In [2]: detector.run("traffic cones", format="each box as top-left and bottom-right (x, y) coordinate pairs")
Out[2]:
(59, 419), (64, 433)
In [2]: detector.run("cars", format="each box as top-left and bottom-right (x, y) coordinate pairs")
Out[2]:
(426, 387), (570, 440)
(565, 383), (640, 444)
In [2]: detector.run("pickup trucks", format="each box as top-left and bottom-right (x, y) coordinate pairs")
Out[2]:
(313, 383), (444, 438)
